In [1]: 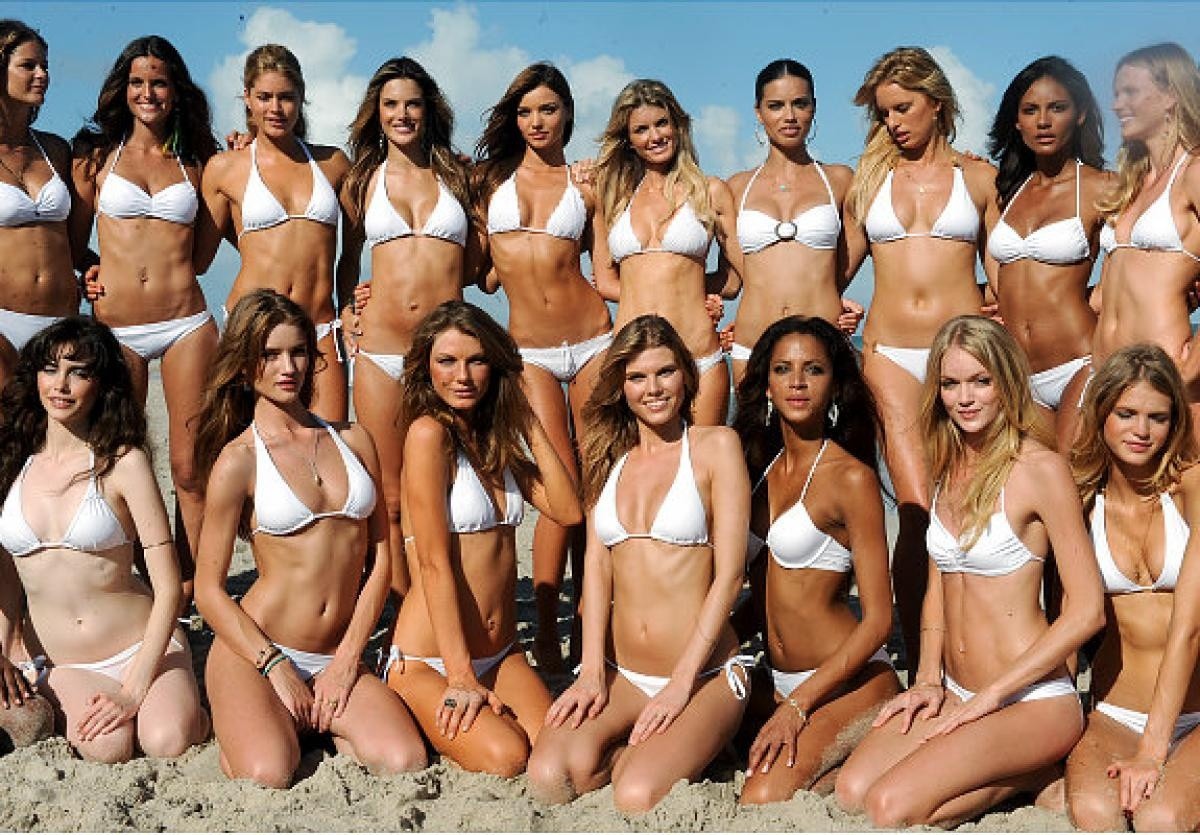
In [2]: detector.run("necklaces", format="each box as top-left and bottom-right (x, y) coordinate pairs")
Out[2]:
(763, 167), (801, 194)
(266, 427), (324, 487)
(897, 164), (944, 193)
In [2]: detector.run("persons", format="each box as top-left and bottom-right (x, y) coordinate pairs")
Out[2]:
(727, 316), (901, 805)
(589, 80), (869, 433)
(528, 312), (761, 817)
(0, 319), (212, 763)
(833, 46), (998, 667)
(1043, 343), (1200, 833)
(0, 18), (725, 702)
(702, 55), (987, 390)
(381, 299), (583, 780)
(978, 51), (1121, 674)
(1094, 44), (1199, 460)
(184, 288), (429, 790)
(833, 314), (1107, 829)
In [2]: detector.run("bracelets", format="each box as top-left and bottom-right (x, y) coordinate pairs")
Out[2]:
(788, 696), (807, 721)
(256, 644), (280, 671)
(262, 656), (291, 676)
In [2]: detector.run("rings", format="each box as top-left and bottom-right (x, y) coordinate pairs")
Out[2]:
(328, 701), (337, 708)
(445, 699), (455, 710)
